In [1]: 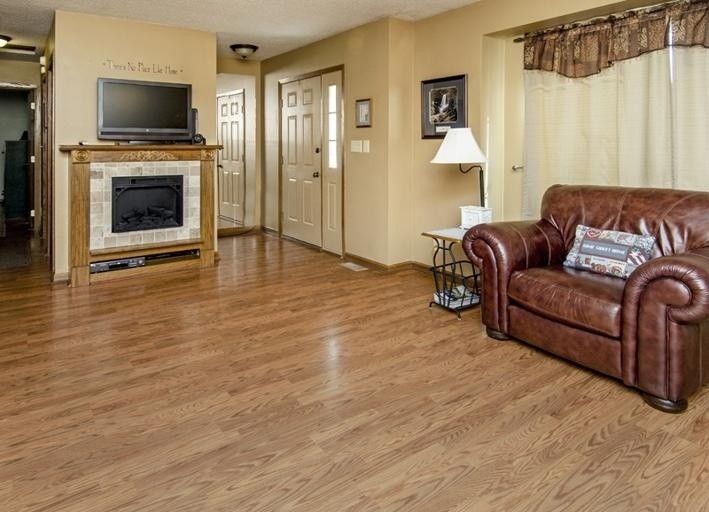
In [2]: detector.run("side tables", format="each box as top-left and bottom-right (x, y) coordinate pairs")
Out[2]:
(419, 227), (482, 320)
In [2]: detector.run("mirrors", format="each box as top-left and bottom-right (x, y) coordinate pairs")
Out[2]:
(216, 73), (257, 231)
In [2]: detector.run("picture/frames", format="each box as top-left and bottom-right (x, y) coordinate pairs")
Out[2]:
(355, 98), (372, 127)
(420, 73), (468, 139)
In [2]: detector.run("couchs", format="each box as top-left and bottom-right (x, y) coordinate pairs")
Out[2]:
(460, 185), (709, 414)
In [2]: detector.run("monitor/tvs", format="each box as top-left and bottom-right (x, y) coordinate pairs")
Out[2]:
(97, 78), (193, 144)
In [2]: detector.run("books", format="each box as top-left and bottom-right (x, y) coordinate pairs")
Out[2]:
(433, 284), (480, 309)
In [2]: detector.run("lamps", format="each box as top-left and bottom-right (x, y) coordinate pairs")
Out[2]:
(430, 128), (485, 209)
(0, 34), (11, 48)
(229, 43), (258, 59)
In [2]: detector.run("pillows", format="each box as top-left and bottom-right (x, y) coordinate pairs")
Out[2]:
(563, 224), (656, 283)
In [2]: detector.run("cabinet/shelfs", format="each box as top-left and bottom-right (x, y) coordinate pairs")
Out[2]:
(2, 141), (33, 237)
